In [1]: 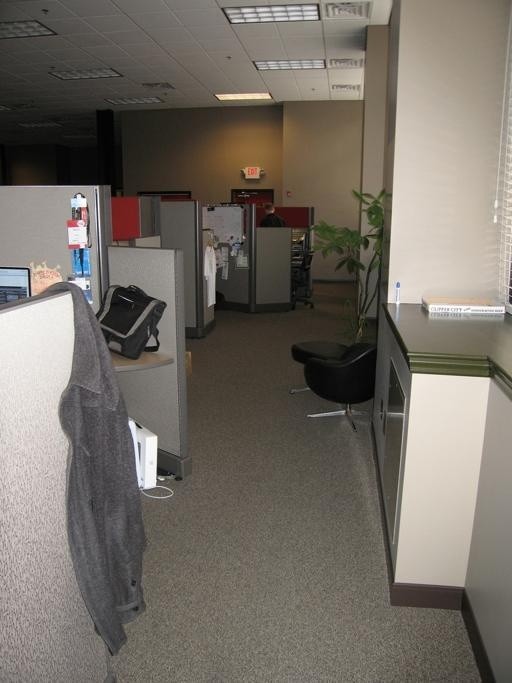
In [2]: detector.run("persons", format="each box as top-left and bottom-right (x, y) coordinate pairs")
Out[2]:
(260, 202), (288, 227)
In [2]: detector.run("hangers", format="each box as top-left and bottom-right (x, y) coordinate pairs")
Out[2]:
(208, 231), (213, 245)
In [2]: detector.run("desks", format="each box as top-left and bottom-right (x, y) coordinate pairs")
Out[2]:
(110, 351), (173, 372)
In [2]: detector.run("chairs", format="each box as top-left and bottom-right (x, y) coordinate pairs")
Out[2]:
(292, 252), (314, 310)
(305, 344), (376, 433)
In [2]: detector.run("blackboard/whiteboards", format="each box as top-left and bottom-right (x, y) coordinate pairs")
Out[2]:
(202, 204), (244, 245)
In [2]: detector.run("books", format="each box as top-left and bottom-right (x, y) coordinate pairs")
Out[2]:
(428, 311), (504, 321)
(421, 296), (506, 313)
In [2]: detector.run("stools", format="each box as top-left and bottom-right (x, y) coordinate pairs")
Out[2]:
(289, 342), (349, 394)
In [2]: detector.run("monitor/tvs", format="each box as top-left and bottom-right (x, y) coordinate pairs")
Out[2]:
(133, 233), (161, 248)
(0, 267), (31, 306)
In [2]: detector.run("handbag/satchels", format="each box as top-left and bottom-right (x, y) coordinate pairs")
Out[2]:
(96, 284), (167, 359)
